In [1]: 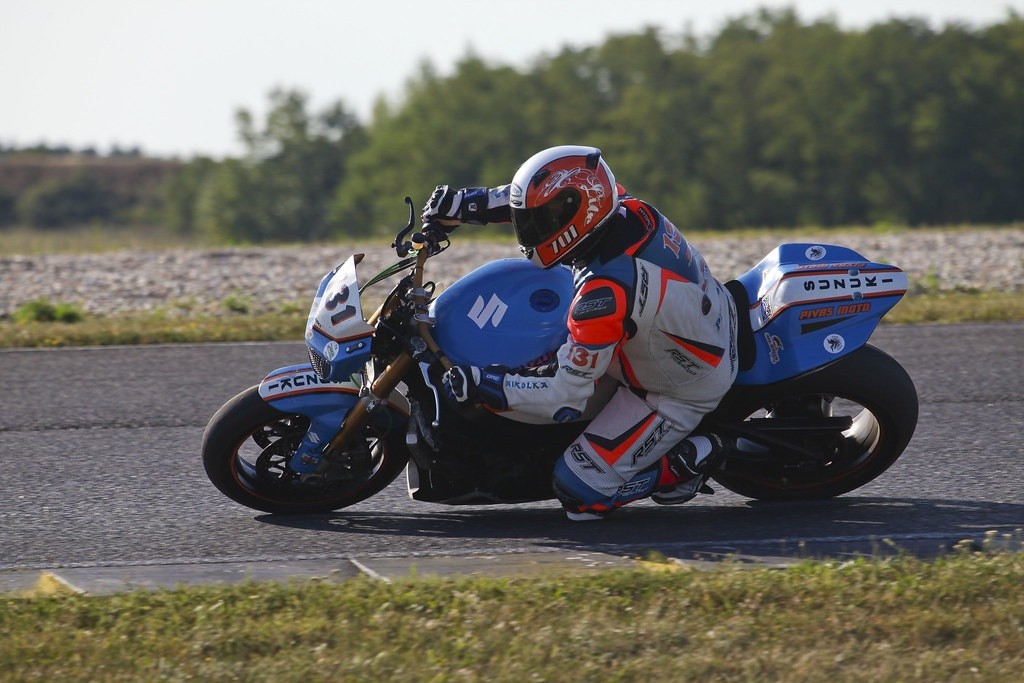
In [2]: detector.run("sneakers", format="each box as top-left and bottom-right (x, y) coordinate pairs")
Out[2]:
(652, 433), (727, 505)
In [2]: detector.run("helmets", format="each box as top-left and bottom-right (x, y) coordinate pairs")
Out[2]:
(509, 145), (620, 270)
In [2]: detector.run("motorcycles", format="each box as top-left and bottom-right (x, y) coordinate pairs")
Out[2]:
(202, 183), (922, 518)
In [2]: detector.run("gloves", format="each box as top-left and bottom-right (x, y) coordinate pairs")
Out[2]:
(442, 362), (515, 411)
(420, 185), (489, 226)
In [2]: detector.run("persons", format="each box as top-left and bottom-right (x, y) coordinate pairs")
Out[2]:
(419, 142), (744, 525)
